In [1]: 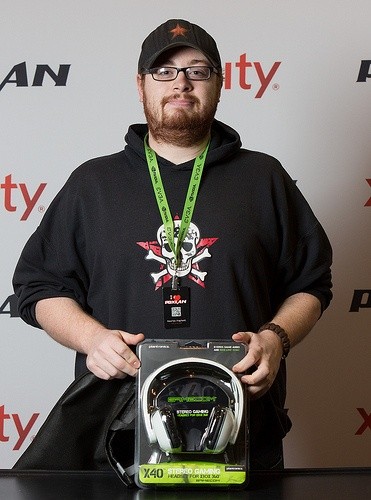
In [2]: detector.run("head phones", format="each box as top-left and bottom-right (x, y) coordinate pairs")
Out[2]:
(140, 357), (244, 454)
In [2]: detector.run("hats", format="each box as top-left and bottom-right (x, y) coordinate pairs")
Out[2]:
(138, 19), (219, 76)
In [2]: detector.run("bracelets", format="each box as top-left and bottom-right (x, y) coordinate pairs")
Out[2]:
(259, 322), (289, 361)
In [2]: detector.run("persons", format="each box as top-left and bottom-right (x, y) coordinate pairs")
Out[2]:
(12, 19), (334, 469)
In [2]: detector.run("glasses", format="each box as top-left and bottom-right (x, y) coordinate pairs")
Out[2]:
(140, 66), (219, 81)
